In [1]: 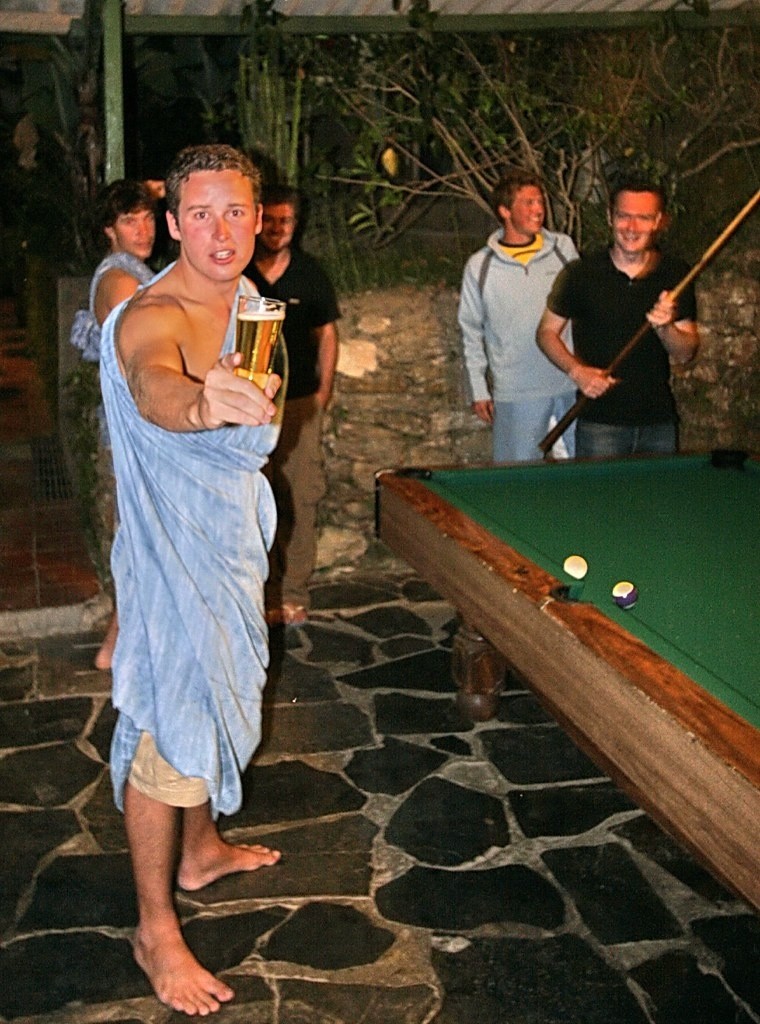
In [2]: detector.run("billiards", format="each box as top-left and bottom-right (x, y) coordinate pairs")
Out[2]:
(562, 554), (590, 582)
(610, 578), (641, 611)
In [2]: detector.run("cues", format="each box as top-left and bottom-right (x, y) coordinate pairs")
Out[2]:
(534, 184), (760, 457)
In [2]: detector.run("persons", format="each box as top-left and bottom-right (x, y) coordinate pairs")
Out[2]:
(241, 184), (342, 624)
(536, 178), (699, 458)
(98, 146), (288, 1014)
(458, 177), (580, 464)
(68, 180), (158, 670)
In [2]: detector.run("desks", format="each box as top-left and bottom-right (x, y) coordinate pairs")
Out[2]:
(375, 449), (760, 916)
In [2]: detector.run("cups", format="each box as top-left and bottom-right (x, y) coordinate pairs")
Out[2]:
(234, 295), (287, 392)
